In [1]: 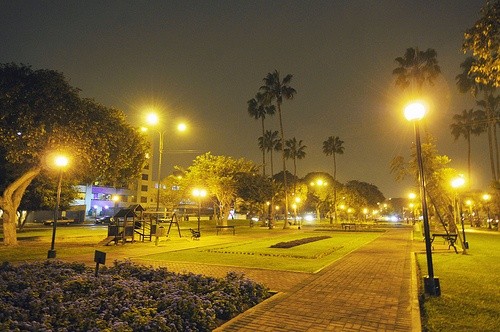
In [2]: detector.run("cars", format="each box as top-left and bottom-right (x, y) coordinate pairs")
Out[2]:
(43, 216), (75, 226)
(158, 216), (172, 223)
(96, 216), (111, 225)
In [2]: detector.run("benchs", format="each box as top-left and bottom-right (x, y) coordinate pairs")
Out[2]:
(216, 226), (236, 236)
(430, 233), (458, 249)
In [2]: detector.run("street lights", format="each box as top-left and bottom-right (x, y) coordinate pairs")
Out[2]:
(451, 177), (470, 249)
(192, 189), (207, 231)
(403, 101), (443, 295)
(146, 112), (188, 224)
(45, 156), (68, 257)
